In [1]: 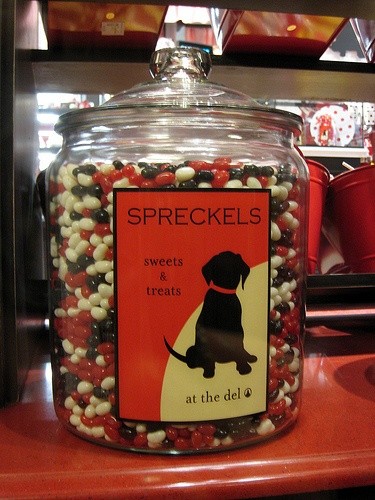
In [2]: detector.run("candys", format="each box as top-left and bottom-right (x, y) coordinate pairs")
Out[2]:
(46, 155), (302, 452)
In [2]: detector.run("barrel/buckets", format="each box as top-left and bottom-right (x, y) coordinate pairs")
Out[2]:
(328, 166), (375, 275)
(304, 158), (333, 275)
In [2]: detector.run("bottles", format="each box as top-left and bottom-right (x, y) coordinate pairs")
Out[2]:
(45, 48), (309, 455)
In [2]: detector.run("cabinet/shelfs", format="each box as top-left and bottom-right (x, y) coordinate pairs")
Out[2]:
(0, 0), (375, 499)
